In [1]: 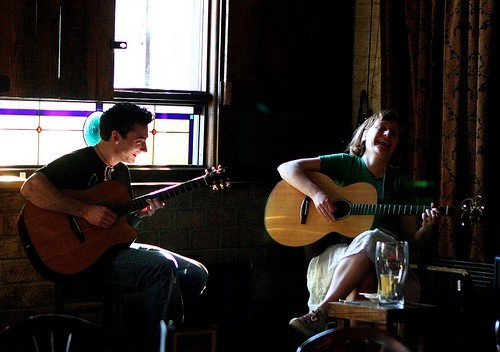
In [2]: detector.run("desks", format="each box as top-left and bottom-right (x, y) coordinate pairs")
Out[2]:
(328, 302), (427, 352)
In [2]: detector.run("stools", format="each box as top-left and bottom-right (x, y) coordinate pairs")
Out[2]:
(54, 285), (132, 337)
(172, 325), (222, 352)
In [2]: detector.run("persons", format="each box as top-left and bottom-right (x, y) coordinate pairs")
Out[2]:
(20, 103), (210, 352)
(276, 110), (442, 337)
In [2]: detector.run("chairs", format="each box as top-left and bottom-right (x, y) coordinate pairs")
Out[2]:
(295, 326), (412, 352)
(0, 313), (127, 352)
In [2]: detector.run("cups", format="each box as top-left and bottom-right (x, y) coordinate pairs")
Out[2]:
(375, 241), (409, 308)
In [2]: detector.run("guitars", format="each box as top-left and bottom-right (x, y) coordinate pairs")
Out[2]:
(14, 162), (233, 282)
(264, 171), (485, 257)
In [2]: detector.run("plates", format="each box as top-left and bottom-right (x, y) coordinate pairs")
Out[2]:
(359, 292), (378, 301)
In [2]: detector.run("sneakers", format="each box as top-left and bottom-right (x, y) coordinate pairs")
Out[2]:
(287, 307), (330, 338)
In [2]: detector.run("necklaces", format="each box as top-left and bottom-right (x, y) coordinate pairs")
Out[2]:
(96, 144), (112, 167)
